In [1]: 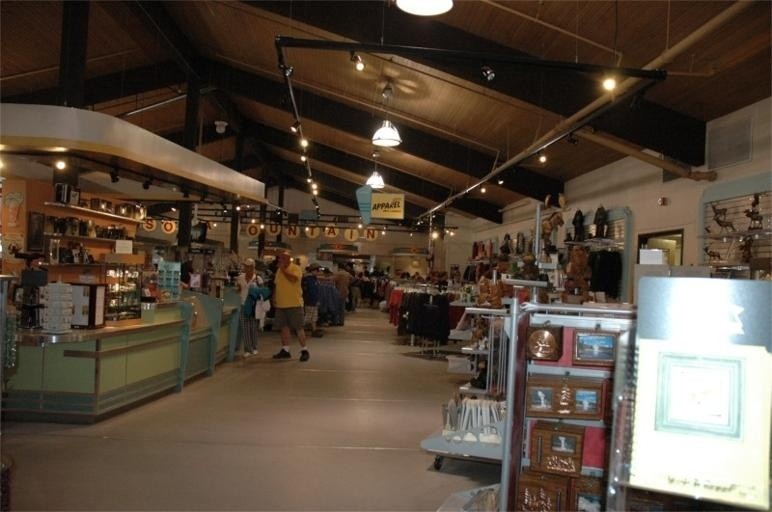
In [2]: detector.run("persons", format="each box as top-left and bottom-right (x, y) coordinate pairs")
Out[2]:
(180, 251), (355, 361)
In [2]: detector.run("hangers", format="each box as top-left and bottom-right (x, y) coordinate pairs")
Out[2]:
(394, 283), (464, 299)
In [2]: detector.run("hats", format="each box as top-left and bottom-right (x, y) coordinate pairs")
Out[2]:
(240, 258), (256, 268)
(308, 263), (321, 272)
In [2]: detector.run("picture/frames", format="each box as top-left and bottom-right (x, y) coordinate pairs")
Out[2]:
(571, 328), (619, 369)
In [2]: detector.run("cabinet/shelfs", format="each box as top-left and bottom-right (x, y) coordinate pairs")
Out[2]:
(455, 304), (511, 399)
(41, 200), (147, 272)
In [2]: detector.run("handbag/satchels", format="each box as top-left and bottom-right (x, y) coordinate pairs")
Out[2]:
(256, 300), (270, 320)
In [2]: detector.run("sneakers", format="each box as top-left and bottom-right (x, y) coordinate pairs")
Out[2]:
(273, 348), (290, 358)
(312, 330), (324, 337)
(300, 350), (309, 360)
(243, 349), (258, 358)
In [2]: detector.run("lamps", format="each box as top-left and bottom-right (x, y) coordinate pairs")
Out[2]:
(364, 83), (404, 190)
